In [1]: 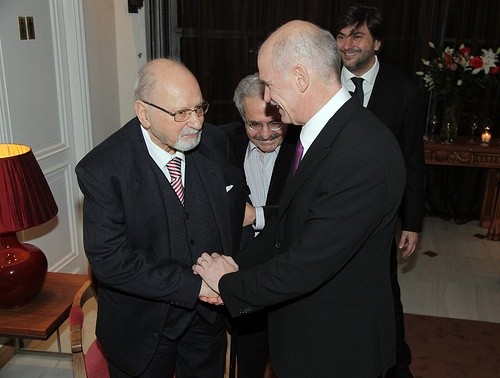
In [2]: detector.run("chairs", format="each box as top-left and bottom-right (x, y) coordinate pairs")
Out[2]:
(69, 279), (110, 378)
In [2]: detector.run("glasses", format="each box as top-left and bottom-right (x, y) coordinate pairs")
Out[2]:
(242, 112), (283, 131)
(142, 100), (209, 122)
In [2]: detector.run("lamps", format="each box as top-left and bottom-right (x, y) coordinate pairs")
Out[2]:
(0, 142), (58, 312)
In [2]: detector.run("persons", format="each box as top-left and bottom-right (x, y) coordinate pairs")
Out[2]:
(191, 20), (405, 378)
(227, 72), (300, 378)
(332, 7), (426, 378)
(74, 57), (233, 378)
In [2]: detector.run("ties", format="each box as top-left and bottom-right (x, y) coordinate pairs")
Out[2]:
(293, 140), (303, 176)
(350, 77), (365, 107)
(165, 157), (184, 207)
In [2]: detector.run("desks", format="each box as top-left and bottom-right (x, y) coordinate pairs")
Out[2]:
(0, 271), (91, 352)
(424, 137), (500, 239)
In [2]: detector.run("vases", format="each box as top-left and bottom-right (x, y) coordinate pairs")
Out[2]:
(438, 107), (463, 146)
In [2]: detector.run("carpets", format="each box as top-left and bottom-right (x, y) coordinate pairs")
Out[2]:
(404, 313), (500, 378)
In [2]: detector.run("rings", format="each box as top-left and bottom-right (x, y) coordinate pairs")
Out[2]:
(201, 259), (205, 264)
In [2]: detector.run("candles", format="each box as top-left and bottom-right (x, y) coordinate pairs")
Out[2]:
(480, 126), (492, 148)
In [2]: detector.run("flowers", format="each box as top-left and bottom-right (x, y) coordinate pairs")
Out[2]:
(414, 43), (500, 113)
